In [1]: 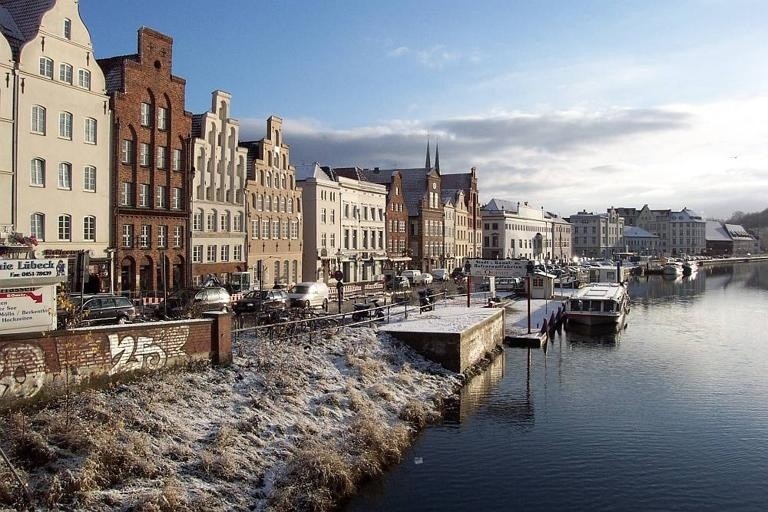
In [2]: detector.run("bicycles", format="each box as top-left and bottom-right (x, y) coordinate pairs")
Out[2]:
(257, 303), (340, 345)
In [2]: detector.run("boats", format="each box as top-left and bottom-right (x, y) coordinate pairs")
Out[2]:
(564, 260), (631, 325)
(661, 261), (698, 275)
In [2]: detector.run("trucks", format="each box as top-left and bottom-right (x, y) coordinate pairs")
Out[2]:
(433, 269), (449, 283)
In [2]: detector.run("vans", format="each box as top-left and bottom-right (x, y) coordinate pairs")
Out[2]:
(287, 282), (329, 309)
(402, 270), (423, 286)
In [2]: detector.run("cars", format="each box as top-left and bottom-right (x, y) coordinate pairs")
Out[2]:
(452, 268), (464, 277)
(233, 291), (291, 317)
(648, 254), (711, 262)
(422, 273), (434, 285)
(533, 259), (613, 285)
(387, 276), (411, 290)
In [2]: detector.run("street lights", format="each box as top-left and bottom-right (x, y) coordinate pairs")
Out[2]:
(335, 249), (344, 313)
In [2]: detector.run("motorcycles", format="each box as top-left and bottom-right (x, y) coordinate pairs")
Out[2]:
(352, 297), (384, 321)
(419, 288), (434, 312)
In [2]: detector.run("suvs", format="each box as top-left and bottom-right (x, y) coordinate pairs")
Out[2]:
(480, 278), (520, 291)
(154, 286), (233, 321)
(57, 294), (136, 328)
